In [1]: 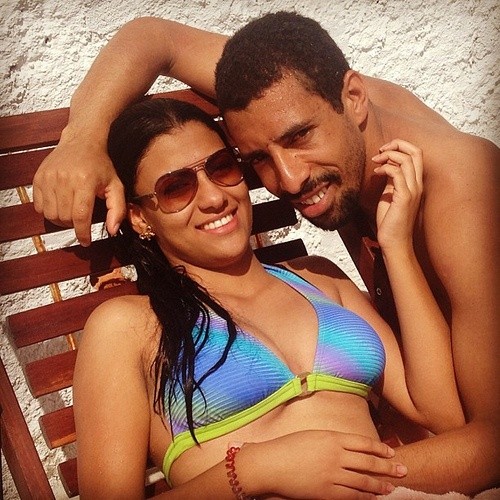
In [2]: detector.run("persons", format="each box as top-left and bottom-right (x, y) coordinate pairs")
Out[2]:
(72, 98), (471, 500)
(33, 11), (500, 499)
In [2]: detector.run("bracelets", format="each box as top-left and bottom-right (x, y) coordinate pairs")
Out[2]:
(225, 447), (256, 500)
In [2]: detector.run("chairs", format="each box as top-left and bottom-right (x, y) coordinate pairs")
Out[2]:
(0, 88), (400, 500)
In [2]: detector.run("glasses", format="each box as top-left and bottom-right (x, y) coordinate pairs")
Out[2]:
(134, 142), (244, 212)
